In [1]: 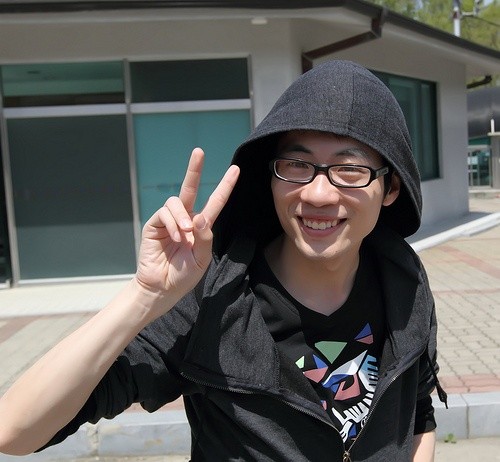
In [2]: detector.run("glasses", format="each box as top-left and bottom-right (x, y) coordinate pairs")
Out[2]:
(269, 156), (389, 188)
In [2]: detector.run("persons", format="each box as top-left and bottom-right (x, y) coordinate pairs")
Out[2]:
(0, 56), (448, 461)
